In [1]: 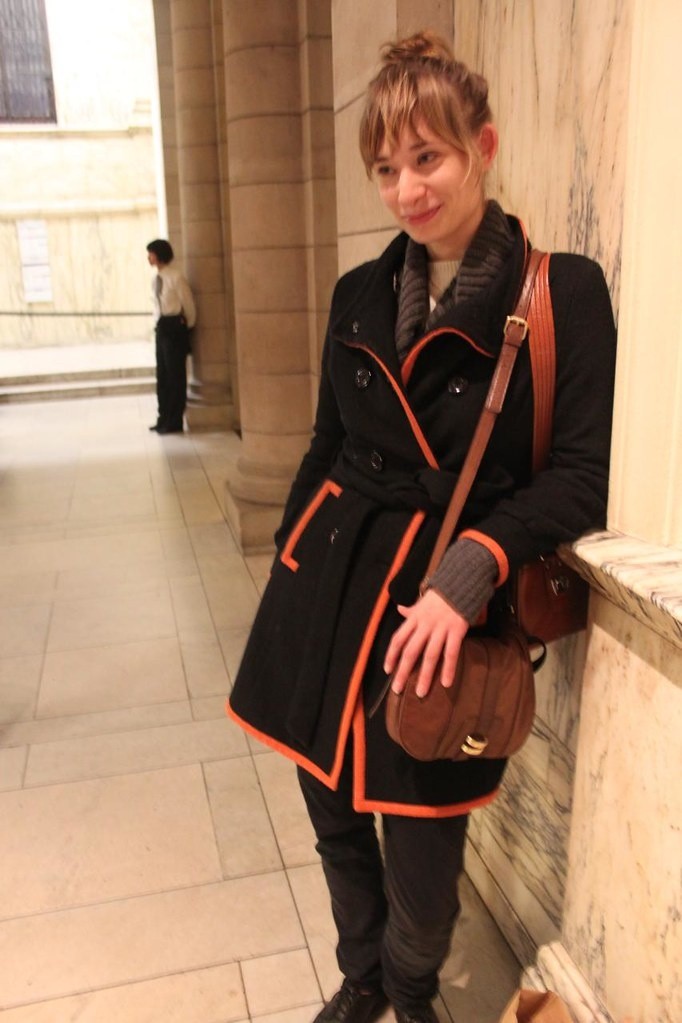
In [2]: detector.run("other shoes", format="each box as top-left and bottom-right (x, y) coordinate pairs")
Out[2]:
(311, 985), (439, 1023)
(149, 421), (184, 434)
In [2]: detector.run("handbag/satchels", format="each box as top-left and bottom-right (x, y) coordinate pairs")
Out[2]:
(385, 639), (536, 760)
(509, 561), (587, 648)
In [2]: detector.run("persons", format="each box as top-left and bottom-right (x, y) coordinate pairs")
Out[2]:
(227, 29), (616, 1023)
(146, 238), (199, 435)
